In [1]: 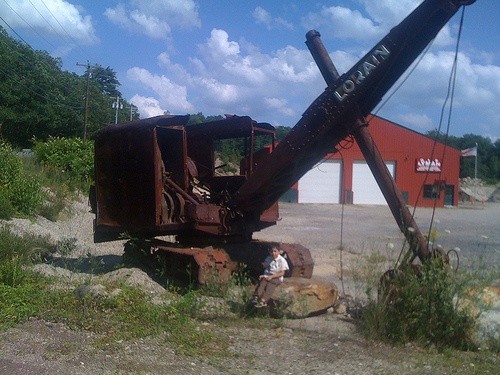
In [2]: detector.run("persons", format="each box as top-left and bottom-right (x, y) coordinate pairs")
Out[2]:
(250, 244), (290, 308)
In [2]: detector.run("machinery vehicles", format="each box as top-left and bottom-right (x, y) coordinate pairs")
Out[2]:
(89, 1), (478, 296)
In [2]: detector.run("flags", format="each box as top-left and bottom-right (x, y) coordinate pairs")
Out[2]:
(461, 146), (476, 157)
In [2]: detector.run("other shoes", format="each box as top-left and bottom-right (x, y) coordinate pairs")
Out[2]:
(254, 300), (267, 307)
(251, 296), (258, 303)
